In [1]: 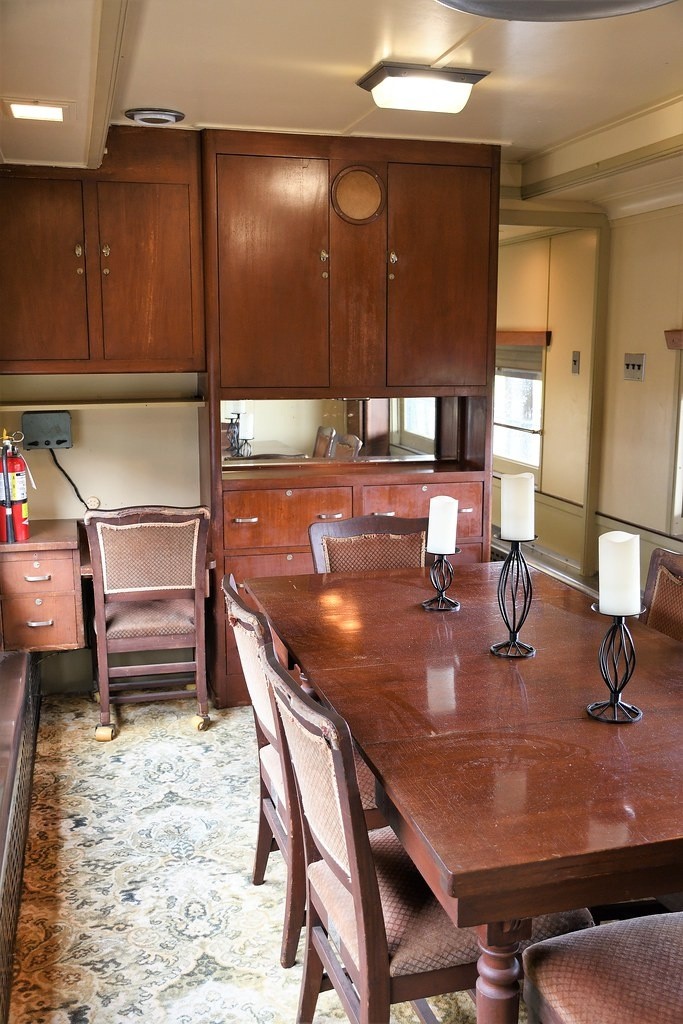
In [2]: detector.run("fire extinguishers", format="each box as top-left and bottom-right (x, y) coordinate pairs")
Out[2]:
(0, 429), (29, 543)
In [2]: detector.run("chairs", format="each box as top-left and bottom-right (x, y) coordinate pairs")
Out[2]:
(85, 505), (211, 739)
(257, 647), (598, 1024)
(328, 434), (363, 459)
(222, 573), (389, 969)
(307, 516), (434, 576)
(640, 548), (683, 642)
(312, 426), (336, 458)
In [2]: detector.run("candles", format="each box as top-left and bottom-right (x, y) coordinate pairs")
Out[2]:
(599, 531), (640, 614)
(426, 496), (458, 554)
(499, 473), (535, 541)
(239, 411), (252, 437)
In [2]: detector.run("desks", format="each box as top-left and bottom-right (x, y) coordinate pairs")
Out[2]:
(220, 440), (308, 462)
(242, 558), (683, 1024)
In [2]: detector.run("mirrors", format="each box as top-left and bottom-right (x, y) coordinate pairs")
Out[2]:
(220, 397), (436, 471)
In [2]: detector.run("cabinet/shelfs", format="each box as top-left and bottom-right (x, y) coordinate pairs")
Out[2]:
(0, 519), (83, 651)
(0, 122), (204, 376)
(199, 129), (499, 709)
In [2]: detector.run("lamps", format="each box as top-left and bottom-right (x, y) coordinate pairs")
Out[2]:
(356, 61), (491, 114)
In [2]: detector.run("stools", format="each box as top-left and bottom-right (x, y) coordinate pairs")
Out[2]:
(522, 912), (683, 1024)
